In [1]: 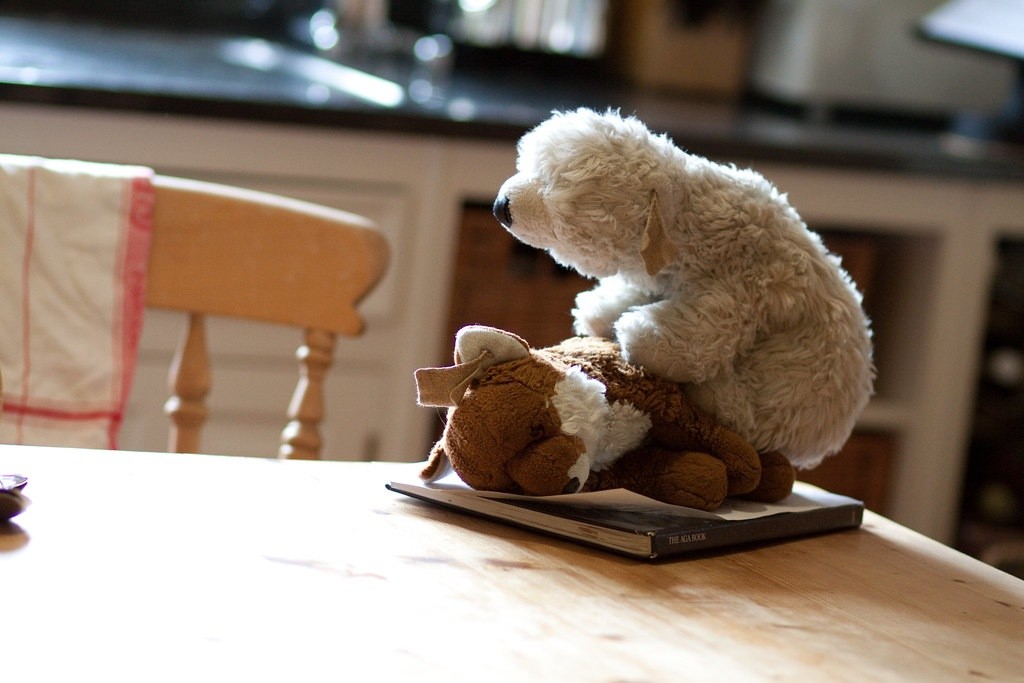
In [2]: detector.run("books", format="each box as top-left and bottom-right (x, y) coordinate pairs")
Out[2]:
(377, 478), (866, 563)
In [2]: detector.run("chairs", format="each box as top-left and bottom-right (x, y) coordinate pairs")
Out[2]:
(141, 174), (391, 460)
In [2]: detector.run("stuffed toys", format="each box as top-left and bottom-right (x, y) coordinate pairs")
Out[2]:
(415, 325), (796, 511)
(492, 105), (876, 471)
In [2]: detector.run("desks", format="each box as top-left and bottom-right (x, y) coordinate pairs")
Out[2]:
(0, 445), (1023, 683)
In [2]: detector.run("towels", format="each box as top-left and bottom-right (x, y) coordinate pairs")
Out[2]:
(1, 151), (157, 450)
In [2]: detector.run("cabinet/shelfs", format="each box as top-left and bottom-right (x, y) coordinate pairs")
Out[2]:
(813, 222), (1024, 583)
